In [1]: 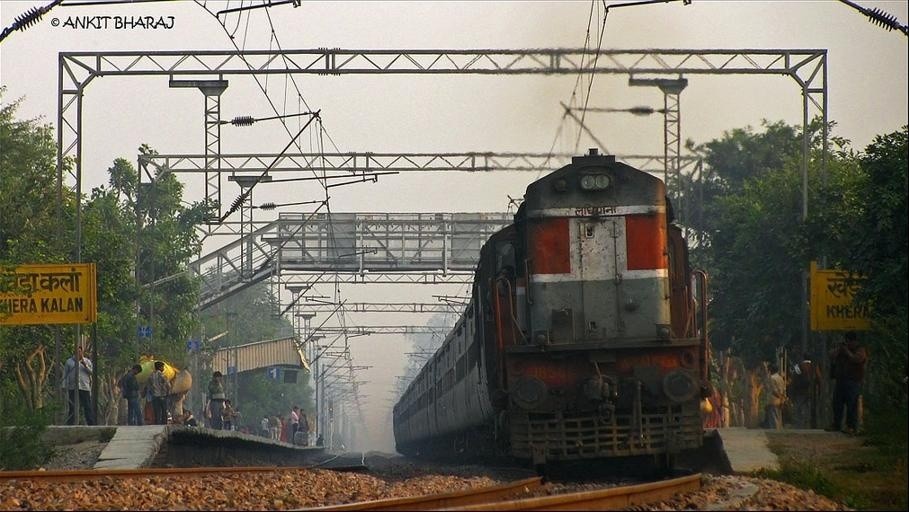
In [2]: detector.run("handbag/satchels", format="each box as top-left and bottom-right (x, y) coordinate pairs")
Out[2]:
(779, 396), (792, 410)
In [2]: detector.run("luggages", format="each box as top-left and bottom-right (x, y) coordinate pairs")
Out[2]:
(292, 428), (307, 446)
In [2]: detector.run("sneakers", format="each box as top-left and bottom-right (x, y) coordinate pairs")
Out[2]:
(841, 425), (856, 434)
(823, 424), (841, 431)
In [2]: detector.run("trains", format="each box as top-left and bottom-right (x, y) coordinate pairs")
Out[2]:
(393, 149), (714, 480)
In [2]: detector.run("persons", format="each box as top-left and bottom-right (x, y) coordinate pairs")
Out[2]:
(63, 345), (323, 447)
(705, 332), (866, 434)
